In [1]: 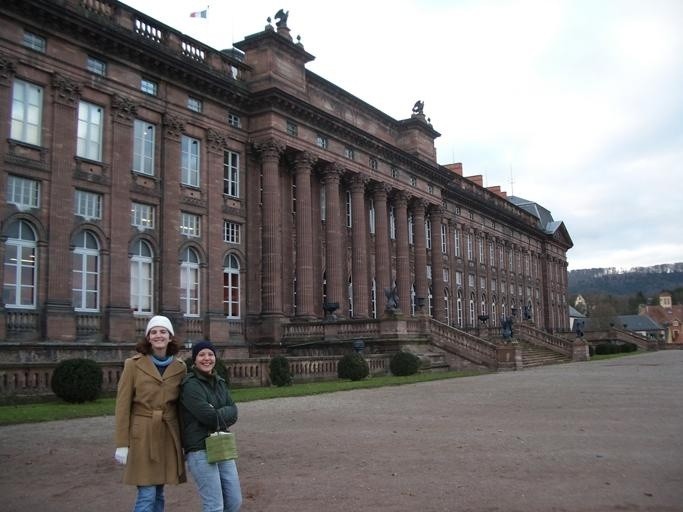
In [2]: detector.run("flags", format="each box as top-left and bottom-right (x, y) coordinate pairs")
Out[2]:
(189, 9), (207, 19)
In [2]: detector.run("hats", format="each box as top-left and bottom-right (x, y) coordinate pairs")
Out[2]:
(145, 316), (174, 337)
(192, 341), (216, 363)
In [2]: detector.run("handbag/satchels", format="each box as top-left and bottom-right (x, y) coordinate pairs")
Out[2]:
(205, 432), (238, 464)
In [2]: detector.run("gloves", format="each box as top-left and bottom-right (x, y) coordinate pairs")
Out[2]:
(115, 447), (128, 465)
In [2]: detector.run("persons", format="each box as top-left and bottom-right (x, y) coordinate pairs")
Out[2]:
(177, 338), (242, 511)
(113, 315), (187, 512)
